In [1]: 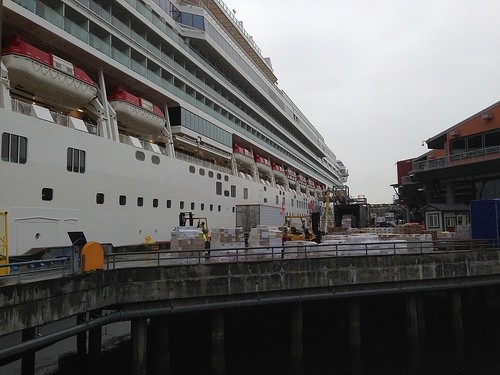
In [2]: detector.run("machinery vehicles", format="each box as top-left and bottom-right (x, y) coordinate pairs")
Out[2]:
(176, 210), (213, 254)
(278, 211), (322, 246)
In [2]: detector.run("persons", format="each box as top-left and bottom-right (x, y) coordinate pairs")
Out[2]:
(197, 222), (212, 254)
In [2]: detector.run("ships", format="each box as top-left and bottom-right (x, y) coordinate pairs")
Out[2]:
(0, 0), (349, 250)
